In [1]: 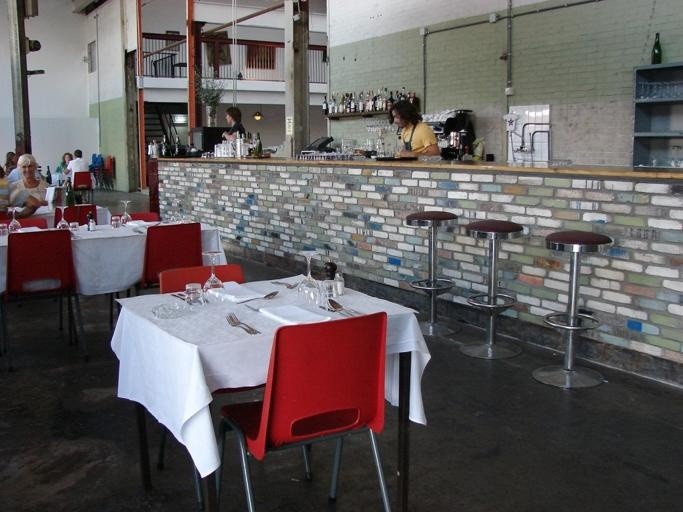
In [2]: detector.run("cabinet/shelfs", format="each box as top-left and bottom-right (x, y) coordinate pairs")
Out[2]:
(630, 62), (683, 169)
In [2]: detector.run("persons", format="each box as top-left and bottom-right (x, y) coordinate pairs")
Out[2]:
(56, 153), (73, 174)
(221, 107), (245, 142)
(0, 152), (51, 219)
(388, 100), (441, 158)
(63, 150), (89, 206)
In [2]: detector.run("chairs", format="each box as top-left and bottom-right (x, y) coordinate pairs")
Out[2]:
(158, 263), (313, 511)
(215, 312), (392, 511)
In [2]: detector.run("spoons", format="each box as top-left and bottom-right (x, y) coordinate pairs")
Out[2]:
(328, 298), (355, 318)
(236, 290), (280, 305)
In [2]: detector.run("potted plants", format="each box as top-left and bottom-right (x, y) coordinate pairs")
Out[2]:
(192, 64), (226, 127)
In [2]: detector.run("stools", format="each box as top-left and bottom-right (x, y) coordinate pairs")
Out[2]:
(406, 211), (462, 337)
(532, 231), (615, 389)
(460, 220), (523, 360)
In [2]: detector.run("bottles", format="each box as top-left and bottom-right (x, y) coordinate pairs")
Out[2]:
(651, 32), (662, 64)
(237, 131), (262, 156)
(45, 165), (52, 184)
(64, 177), (75, 206)
(214, 138), (249, 158)
(87, 211), (96, 232)
(322, 86), (415, 115)
(151, 134), (182, 158)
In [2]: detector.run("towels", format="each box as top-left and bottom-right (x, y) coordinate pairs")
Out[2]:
(259, 305), (331, 325)
(207, 281), (265, 303)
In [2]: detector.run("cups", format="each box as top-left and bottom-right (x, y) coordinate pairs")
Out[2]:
(334, 271), (344, 296)
(636, 80), (683, 99)
(337, 137), (384, 157)
(184, 283), (207, 312)
(671, 145), (681, 168)
(0, 224), (8, 245)
(70, 221), (80, 239)
(111, 217), (122, 232)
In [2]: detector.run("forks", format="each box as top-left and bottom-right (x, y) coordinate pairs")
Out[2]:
(225, 312), (261, 336)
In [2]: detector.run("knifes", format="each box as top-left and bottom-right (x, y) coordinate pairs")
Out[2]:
(319, 305), (347, 317)
(170, 294), (192, 305)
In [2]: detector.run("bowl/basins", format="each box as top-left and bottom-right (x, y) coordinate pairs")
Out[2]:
(152, 302), (188, 319)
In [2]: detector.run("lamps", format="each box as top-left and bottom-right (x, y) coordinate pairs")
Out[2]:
(253, 104), (264, 120)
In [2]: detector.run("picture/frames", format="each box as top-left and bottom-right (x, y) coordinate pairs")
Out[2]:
(166, 30), (180, 51)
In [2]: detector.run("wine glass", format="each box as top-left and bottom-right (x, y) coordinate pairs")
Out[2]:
(202, 251), (224, 306)
(119, 200), (134, 227)
(297, 250), (322, 307)
(56, 205), (69, 230)
(367, 119), (398, 133)
(8, 207), (21, 233)
